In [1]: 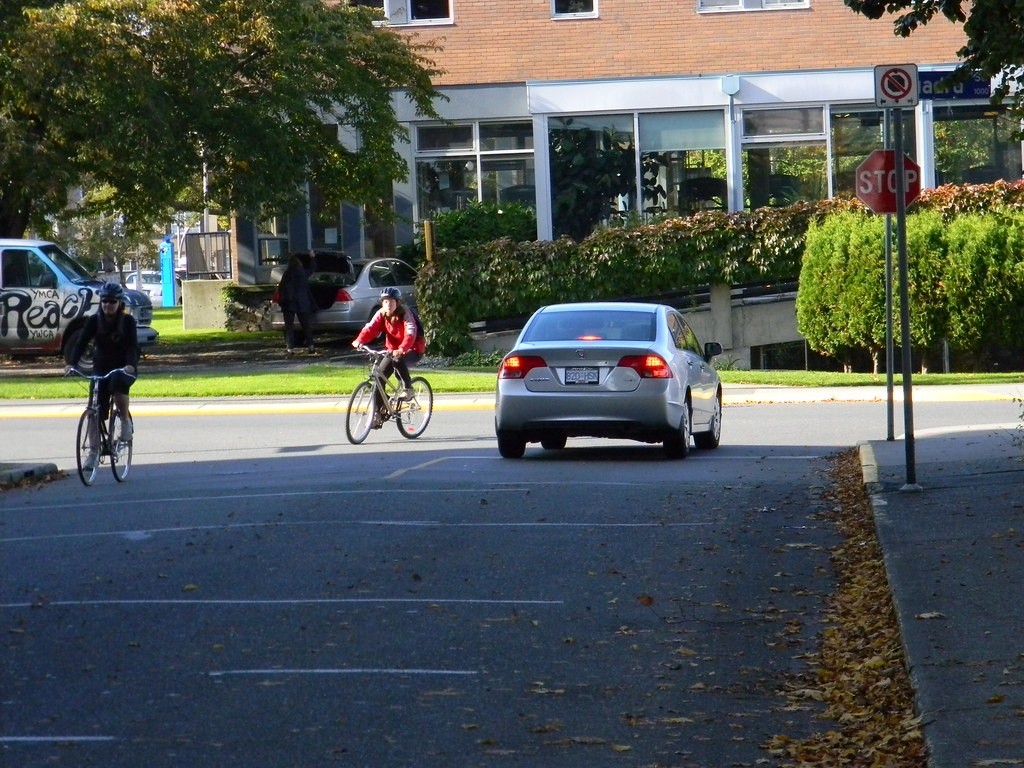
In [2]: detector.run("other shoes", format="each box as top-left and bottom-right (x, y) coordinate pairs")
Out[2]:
(307, 349), (318, 357)
(286, 348), (296, 356)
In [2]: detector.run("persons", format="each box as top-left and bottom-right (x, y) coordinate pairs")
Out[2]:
(277, 265), (321, 354)
(63, 283), (138, 471)
(353, 289), (425, 429)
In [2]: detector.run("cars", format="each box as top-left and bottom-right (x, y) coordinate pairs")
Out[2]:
(120, 269), (185, 306)
(268, 248), (430, 345)
(492, 302), (728, 458)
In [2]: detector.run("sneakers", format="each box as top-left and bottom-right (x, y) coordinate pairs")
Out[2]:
(399, 389), (416, 401)
(371, 413), (383, 430)
(119, 419), (132, 442)
(81, 452), (97, 471)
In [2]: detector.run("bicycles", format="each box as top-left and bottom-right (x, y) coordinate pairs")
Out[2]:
(61, 364), (136, 488)
(343, 345), (435, 444)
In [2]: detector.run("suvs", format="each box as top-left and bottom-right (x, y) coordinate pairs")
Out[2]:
(0, 236), (162, 377)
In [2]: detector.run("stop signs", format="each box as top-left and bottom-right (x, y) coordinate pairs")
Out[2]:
(853, 149), (918, 213)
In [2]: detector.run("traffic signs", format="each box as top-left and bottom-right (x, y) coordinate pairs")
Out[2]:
(915, 69), (991, 101)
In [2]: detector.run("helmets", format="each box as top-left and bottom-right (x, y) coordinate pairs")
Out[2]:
(379, 288), (403, 302)
(99, 282), (125, 300)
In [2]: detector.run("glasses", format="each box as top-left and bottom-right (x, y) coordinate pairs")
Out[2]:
(101, 299), (117, 304)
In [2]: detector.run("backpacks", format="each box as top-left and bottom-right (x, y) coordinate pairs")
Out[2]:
(398, 307), (426, 366)
